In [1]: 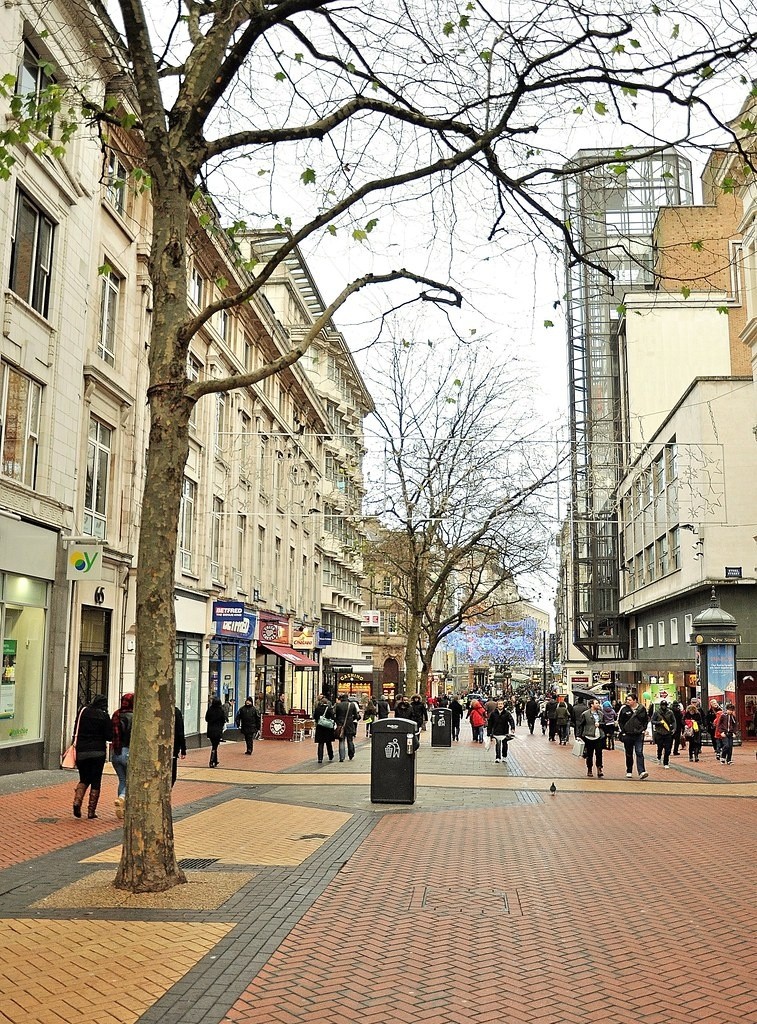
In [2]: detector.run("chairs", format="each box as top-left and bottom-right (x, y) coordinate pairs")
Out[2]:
(293, 719), (316, 742)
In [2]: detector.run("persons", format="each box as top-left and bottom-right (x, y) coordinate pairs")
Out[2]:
(276, 693), (287, 716)
(235, 696), (261, 755)
(312, 682), (739, 779)
(111, 693), (135, 819)
(71, 694), (112, 818)
(255, 691), (275, 714)
(172, 708), (187, 789)
(205, 698), (234, 766)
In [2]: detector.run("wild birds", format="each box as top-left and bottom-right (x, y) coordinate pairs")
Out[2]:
(550, 782), (557, 795)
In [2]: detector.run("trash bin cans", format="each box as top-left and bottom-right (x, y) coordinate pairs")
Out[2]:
(430, 708), (451, 747)
(371, 718), (420, 805)
(652, 721), (657, 744)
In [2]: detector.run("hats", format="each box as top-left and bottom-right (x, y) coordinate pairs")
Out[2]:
(659, 700), (680, 706)
(381, 695), (386, 699)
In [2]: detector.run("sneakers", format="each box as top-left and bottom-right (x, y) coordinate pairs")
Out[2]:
(626, 772), (649, 780)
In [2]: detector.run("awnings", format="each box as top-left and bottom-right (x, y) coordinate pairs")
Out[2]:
(261, 641), (319, 667)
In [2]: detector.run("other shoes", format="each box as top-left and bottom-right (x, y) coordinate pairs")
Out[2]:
(656, 759), (670, 769)
(548, 736), (567, 747)
(327, 755), (335, 762)
(541, 730), (546, 736)
(688, 755), (701, 763)
(453, 735), (484, 744)
(245, 751), (251, 755)
(318, 758), (323, 763)
(670, 750), (680, 756)
(348, 753), (355, 760)
(495, 757), (507, 763)
(338, 757), (346, 763)
(528, 729), (535, 735)
(715, 750), (735, 765)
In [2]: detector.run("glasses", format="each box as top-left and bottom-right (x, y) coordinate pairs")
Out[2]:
(692, 702), (697, 704)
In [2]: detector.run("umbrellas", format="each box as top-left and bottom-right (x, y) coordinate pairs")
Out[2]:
(571, 690), (597, 700)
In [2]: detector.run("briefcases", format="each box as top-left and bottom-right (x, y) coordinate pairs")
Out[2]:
(571, 738), (585, 756)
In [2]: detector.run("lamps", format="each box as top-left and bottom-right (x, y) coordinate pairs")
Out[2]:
(679, 524), (697, 535)
(307, 509), (320, 514)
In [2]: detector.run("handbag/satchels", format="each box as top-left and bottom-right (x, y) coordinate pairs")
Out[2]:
(670, 724), (674, 735)
(362, 716), (372, 723)
(318, 707), (334, 728)
(422, 722), (427, 731)
(683, 725), (694, 737)
(62, 745), (78, 769)
(485, 736), (491, 751)
(335, 724), (344, 739)
(618, 730), (627, 742)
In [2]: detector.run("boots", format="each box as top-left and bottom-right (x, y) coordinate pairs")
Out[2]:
(587, 767), (604, 777)
(73, 783), (123, 818)
(209, 749), (219, 768)
